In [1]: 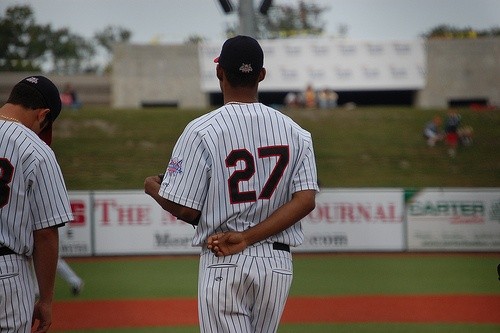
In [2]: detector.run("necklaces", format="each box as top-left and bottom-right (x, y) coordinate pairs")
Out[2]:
(1, 115), (21, 122)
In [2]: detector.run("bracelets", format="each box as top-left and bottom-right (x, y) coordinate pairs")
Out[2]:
(159, 174), (164, 184)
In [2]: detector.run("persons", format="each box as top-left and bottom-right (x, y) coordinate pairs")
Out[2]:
(54, 257), (86, 296)
(145, 35), (322, 333)
(283, 80), (343, 111)
(61, 81), (78, 105)
(421, 109), (475, 158)
(1, 76), (74, 333)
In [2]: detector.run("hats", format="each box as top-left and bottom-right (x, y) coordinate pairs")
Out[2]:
(213, 35), (264, 80)
(15, 75), (62, 146)
